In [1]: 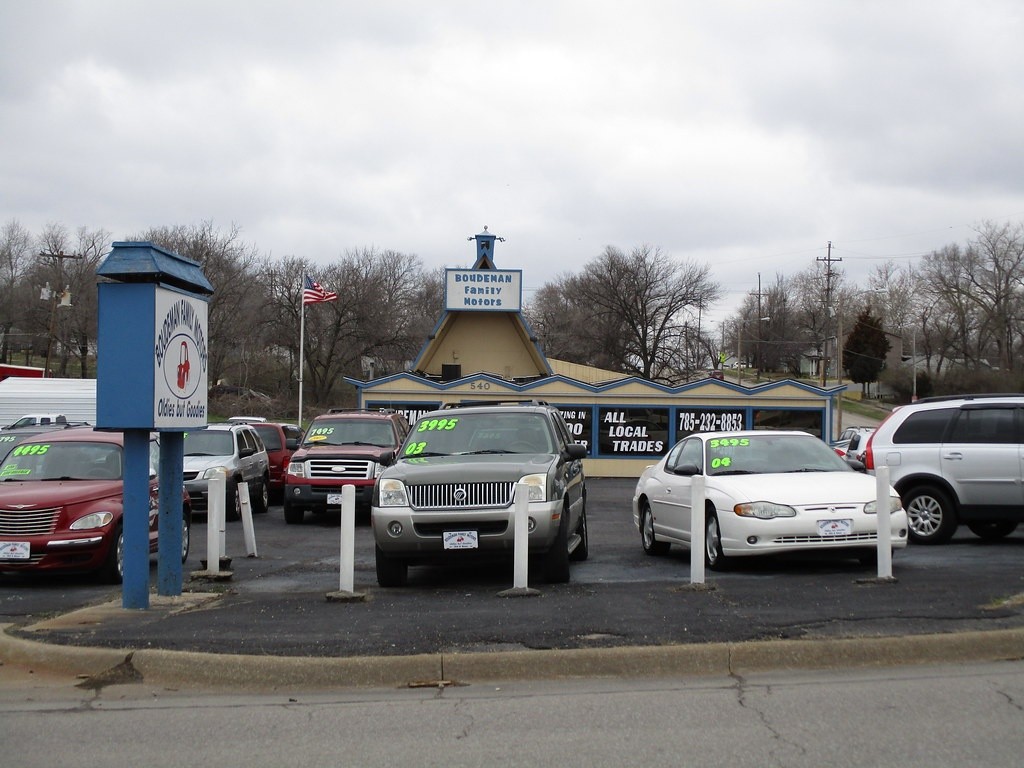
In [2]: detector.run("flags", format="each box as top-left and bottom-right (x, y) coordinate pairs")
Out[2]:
(304, 274), (337, 304)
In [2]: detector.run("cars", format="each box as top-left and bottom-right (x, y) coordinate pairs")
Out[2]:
(0, 412), (194, 585)
(630, 426), (910, 572)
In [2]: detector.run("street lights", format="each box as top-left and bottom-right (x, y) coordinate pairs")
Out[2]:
(736, 316), (771, 386)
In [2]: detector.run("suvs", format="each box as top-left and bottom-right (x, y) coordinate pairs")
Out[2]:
(830, 425), (877, 471)
(372, 396), (590, 587)
(864, 391), (1024, 547)
(280, 407), (411, 525)
(224, 415), (305, 494)
(155, 422), (271, 520)
